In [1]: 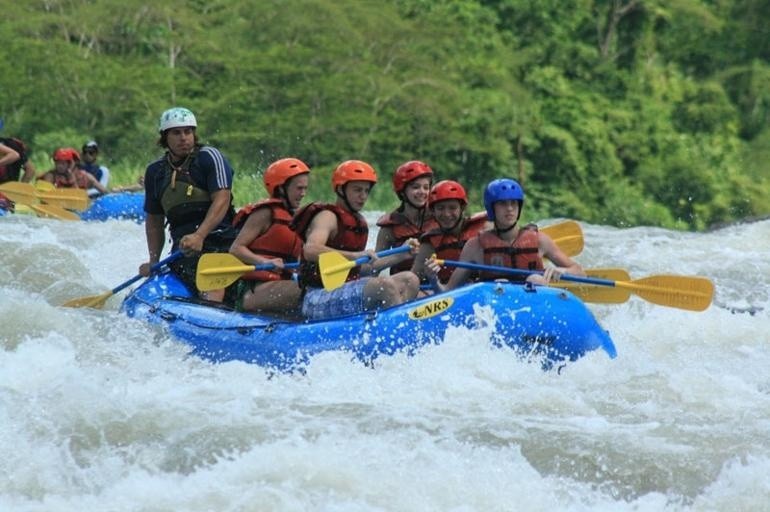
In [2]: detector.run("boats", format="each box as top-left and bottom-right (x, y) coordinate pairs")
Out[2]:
(0, 191), (148, 225)
(118, 272), (617, 379)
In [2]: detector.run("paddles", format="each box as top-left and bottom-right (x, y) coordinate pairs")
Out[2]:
(417, 267), (631, 306)
(57, 250), (184, 309)
(26, 203), (81, 221)
(317, 244), (415, 293)
(433, 257), (715, 322)
(34, 180), (56, 192)
(0, 181), (36, 205)
(36, 188), (88, 212)
(195, 253), (301, 291)
(537, 219), (585, 261)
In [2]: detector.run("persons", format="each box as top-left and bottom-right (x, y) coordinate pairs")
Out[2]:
(409, 180), (495, 284)
(71, 150), (110, 196)
(44, 147), (77, 189)
(376, 161), (433, 275)
(141, 108), (237, 292)
(426, 178), (582, 295)
(0, 142), (21, 167)
(1, 118), (35, 184)
(297, 161), (420, 322)
(78, 141), (109, 199)
(221, 158), (310, 311)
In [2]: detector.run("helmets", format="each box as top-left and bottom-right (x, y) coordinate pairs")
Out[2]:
(395, 161), (434, 191)
(263, 158), (311, 194)
(428, 180), (467, 207)
(333, 160), (378, 191)
(83, 141), (100, 151)
(54, 149), (73, 162)
(159, 108), (198, 132)
(69, 148), (79, 158)
(484, 179), (524, 221)
